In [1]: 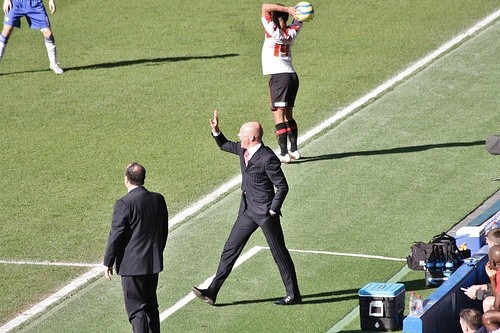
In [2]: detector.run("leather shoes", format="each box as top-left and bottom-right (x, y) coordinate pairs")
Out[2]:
(274, 296), (292, 305)
(191, 287), (215, 305)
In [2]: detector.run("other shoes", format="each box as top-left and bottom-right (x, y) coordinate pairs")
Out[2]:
(288, 151), (301, 159)
(49, 63), (63, 74)
(276, 153), (291, 163)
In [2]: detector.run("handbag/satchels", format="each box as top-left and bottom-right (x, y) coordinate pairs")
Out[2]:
(406, 241), (433, 270)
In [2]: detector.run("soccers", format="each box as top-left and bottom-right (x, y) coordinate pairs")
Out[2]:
(293, 1), (314, 22)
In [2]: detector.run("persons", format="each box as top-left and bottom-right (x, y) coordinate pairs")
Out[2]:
(104, 163), (168, 333)
(459, 228), (500, 333)
(0, 0), (63, 74)
(192, 110), (303, 305)
(261, 3), (315, 164)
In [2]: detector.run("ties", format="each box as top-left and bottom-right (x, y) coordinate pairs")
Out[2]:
(243, 150), (249, 167)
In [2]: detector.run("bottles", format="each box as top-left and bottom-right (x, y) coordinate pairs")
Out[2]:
(492, 222), (499, 229)
(408, 291), (417, 317)
(415, 294), (424, 318)
(424, 259), (454, 287)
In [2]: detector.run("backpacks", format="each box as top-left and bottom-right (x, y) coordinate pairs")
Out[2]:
(426, 232), (464, 286)
